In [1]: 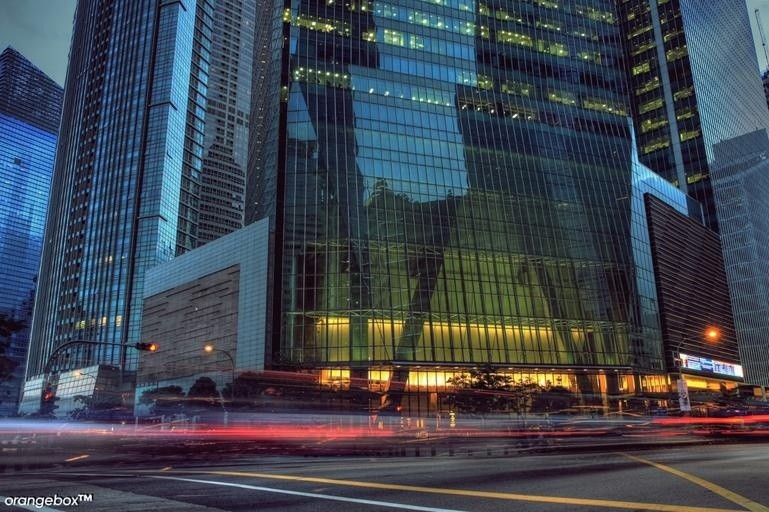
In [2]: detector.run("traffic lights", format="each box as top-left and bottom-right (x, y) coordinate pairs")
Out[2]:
(41, 392), (52, 415)
(50, 396), (60, 415)
(136, 342), (158, 352)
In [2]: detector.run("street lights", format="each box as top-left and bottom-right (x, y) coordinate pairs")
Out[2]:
(203, 345), (235, 407)
(677, 327), (719, 416)
